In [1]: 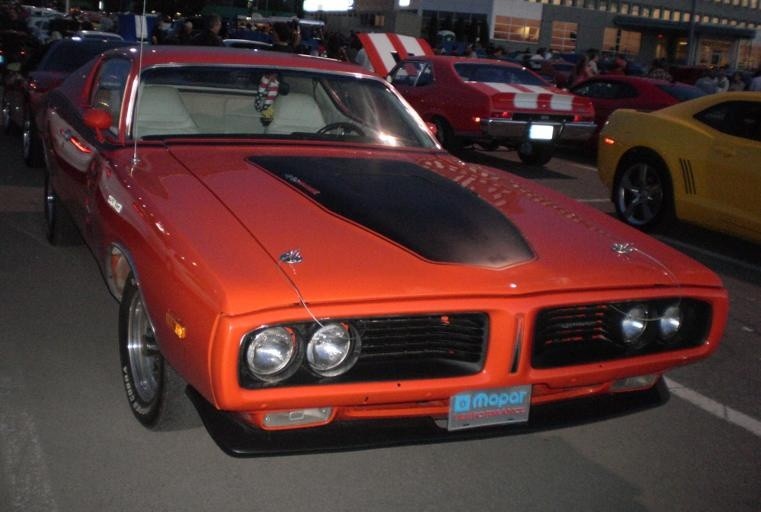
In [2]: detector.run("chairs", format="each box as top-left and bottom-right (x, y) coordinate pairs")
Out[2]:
(95, 85), (330, 137)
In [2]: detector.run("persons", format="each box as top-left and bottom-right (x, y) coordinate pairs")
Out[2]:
(467, 44), (533, 70)
(87, 11), (355, 60)
(586, 52), (761, 94)
(532, 48), (553, 60)
